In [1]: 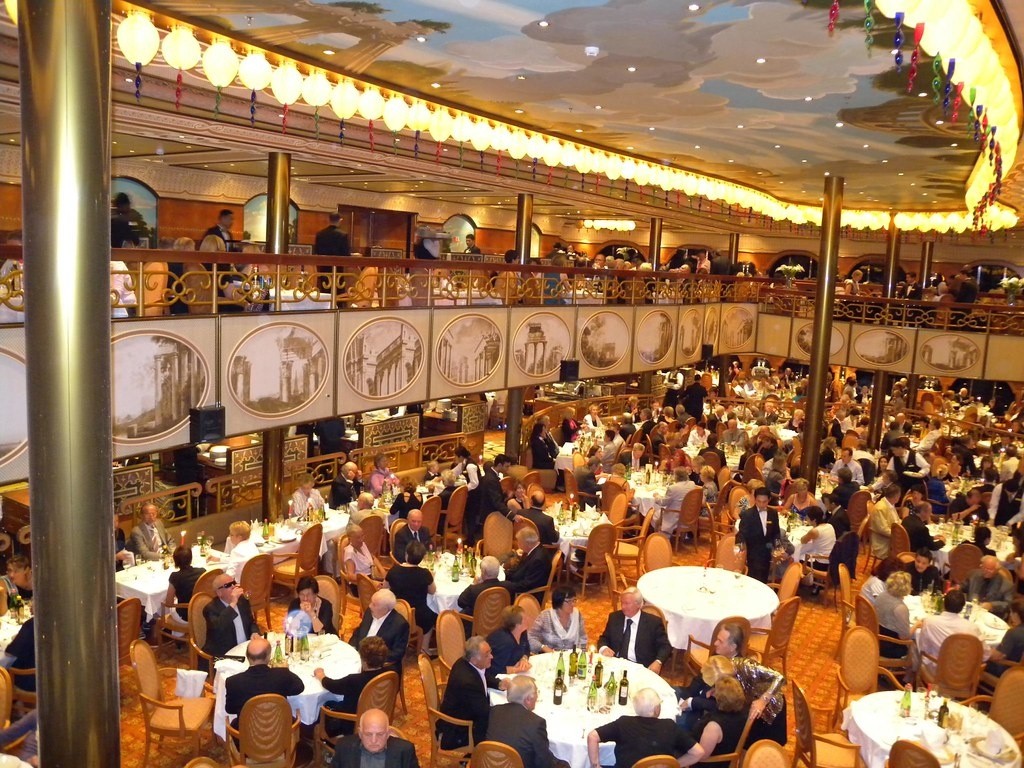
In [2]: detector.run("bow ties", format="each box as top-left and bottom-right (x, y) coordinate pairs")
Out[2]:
(907, 284), (913, 286)
(758, 508), (768, 512)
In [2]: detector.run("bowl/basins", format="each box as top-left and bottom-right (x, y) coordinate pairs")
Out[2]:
(436, 399), (452, 413)
(344, 429), (358, 437)
(209, 444), (231, 466)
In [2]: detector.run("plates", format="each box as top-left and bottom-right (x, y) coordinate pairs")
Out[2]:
(904, 471), (922, 477)
(969, 737), (1018, 763)
(911, 739), (955, 765)
(984, 616), (1007, 630)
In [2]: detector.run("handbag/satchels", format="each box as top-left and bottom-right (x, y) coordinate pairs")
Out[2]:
(801, 553), (814, 586)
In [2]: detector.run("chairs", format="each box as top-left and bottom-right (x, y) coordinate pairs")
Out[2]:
(3, 241), (1017, 330)
(0, 356), (1024, 768)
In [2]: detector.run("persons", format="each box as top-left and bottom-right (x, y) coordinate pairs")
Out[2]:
(0, 361), (1024, 768)
(769, 271), (800, 316)
(841, 264), (979, 331)
(312, 213), (350, 306)
(110, 260), (137, 318)
(463, 234), (480, 254)
(412, 226), (441, 260)
(546, 242), (582, 263)
(167, 209), (270, 316)
(494, 249), (571, 304)
(591, 249), (763, 305)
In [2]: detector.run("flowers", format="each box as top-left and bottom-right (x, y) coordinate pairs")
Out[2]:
(1001, 277), (1022, 290)
(775, 263), (803, 278)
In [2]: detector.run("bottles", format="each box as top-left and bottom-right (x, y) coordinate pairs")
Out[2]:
(199, 531), (206, 557)
(553, 643), (629, 713)
(11, 261), (20, 291)
(378, 480), (401, 512)
(557, 498), (579, 525)
(623, 461), (676, 488)
(951, 514), (961, 545)
(306, 495), (330, 522)
(9, 589), (25, 625)
(259, 271), (272, 289)
(817, 472), (841, 491)
(694, 440), (740, 458)
(937, 698), (949, 729)
(922, 579), (972, 621)
(780, 506), (797, 533)
(572, 422), (611, 458)
(162, 545), (170, 570)
(272, 640), (283, 665)
(898, 683), (912, 718)
(425, 544), (477, 582)
(953, 465), (974, 495)
(346, 418), (352, 431)
(262, 519), (269, 538)
(284, 619), (310, 660)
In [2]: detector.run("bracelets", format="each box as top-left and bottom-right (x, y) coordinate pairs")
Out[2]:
(520, 658), (527, 661)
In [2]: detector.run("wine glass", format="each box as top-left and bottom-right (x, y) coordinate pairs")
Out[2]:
(206, 536), (214, 551)
(968, 702), (988, 735)
(916, 684), (952, 708)
(136, 555), (141, 570)
(123, 559), (131, 575)
(894, 689), (904, 709)
(972, 593), (979, 607)
(170, 544), (176, 556)
(733, 569), (741, 586)
(243, 591), (252, 600)
(191, 542), (198, 555)
(197, 532), (202, 545)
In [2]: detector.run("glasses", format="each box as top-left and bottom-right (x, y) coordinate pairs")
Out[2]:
(564, 599), (578, 604)
(534, 690), (540, 694)
(980, 564), (996, 573)
(219, 580), (236, 589)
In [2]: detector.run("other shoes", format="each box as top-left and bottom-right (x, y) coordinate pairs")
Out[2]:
(141, 622), (164, 631)
(811, 585), (823, 595)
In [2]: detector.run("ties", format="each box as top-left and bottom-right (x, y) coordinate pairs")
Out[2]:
(900, 456), (905, 467)
(618, 619), (633, 659)
(519, 552), (528, 567)
(698, 262), (702, 269)
(414, 532), (417, 540)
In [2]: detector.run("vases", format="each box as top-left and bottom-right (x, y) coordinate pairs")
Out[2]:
(785, 276), (797, 288)
(1003, 287), (1018, 307)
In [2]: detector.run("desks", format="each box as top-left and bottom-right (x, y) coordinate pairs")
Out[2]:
(349, 480), (432, 539)
(510, 648), (686, 768)
(633, 566), (780, 680)
(427, 466), (501, 498)
(225, 285), (337, 314)
(215, 632), (363, 760)
(545, 501), (613, 568)
(919, 518), (1017, 588)
(810, 483), (878, 510)
(554, 430), (623, 489)
(844, 689), (1022, 768)
(557, 286), (603, 305)
(1, 259), (140, 323)
(227, 507), (354, 580)
(604, 472), (689, 533)
(110, 538), (234, 625)
(684, 444), (749, 483)
(416, 552), (507, 612)
(900, 593), (1012, 679)
(734, 509), (824, 559)
(395, 284), (505, 305)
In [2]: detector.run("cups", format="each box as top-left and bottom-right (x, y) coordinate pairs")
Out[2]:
(269, 525), (274, 536)
(318, 630), (325, 637)
(939, 516), (1009, 550)
(716, 564), (724, 573)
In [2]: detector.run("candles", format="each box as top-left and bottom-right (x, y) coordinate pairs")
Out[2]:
(181, 530), (186, 544)
(288, 499), (292, 517)
(926, 680), (931, 698)
(612, 416), (616, 424)
(658, 406), (661, 414)
(457, 536), (462, 551)
(389, 473), (395, 485)
(569, 493), (574, 505)
(710, 364), (714, 370)
(972, 514), (977, 524)
(254, 266), (258, 280)
(477, 452), (483, 463)
(652, 460), (658, 472)
(944, 580), (952, 595)
(590, 644), (594, 662)
